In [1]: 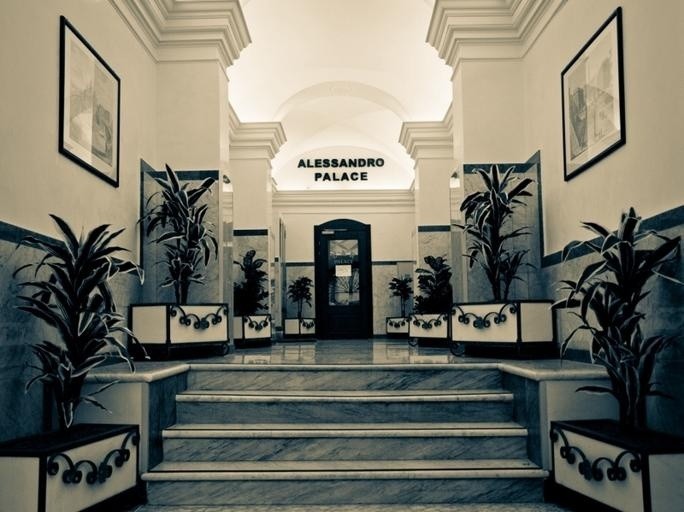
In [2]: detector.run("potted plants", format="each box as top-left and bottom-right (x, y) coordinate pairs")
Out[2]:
(0, 206), (152, 512)
(406, 253), (453, 347)
(447, 163), (556, 361)
(125, 162), (231, 362)
(229, 248), (275, 349)
(544, 203), (683, 512)
(384, 273), (414, 339)
(282, 274), (317, 341)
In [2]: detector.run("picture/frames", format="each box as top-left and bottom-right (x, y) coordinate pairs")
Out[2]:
(556, 6), (627, 185)
(54, 14), (124, 191)
